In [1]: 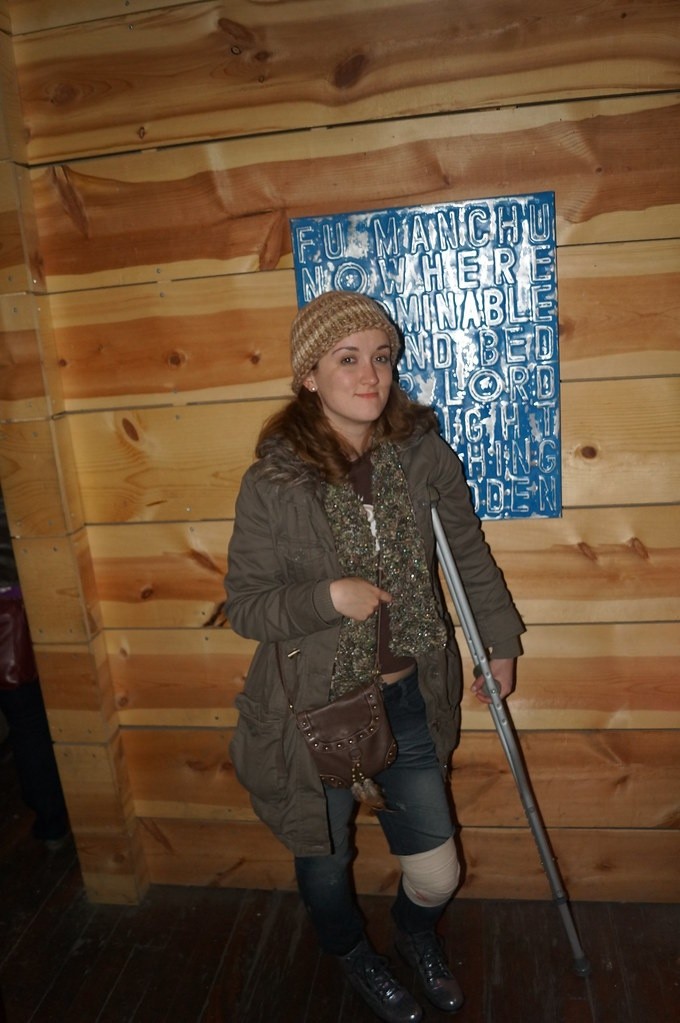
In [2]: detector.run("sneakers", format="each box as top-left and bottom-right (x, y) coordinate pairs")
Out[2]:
(395, 929), (464, 1015)
(334, 938), (423, 1023)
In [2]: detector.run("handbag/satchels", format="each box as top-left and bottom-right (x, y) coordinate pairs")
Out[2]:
(292, 679), (400, 814)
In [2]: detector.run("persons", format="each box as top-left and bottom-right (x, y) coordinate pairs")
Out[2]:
(221, 290), (528, 1022)
(0, 485), (72, 849)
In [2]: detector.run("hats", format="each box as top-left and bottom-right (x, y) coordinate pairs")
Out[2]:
(289, 290), (401, 396)
